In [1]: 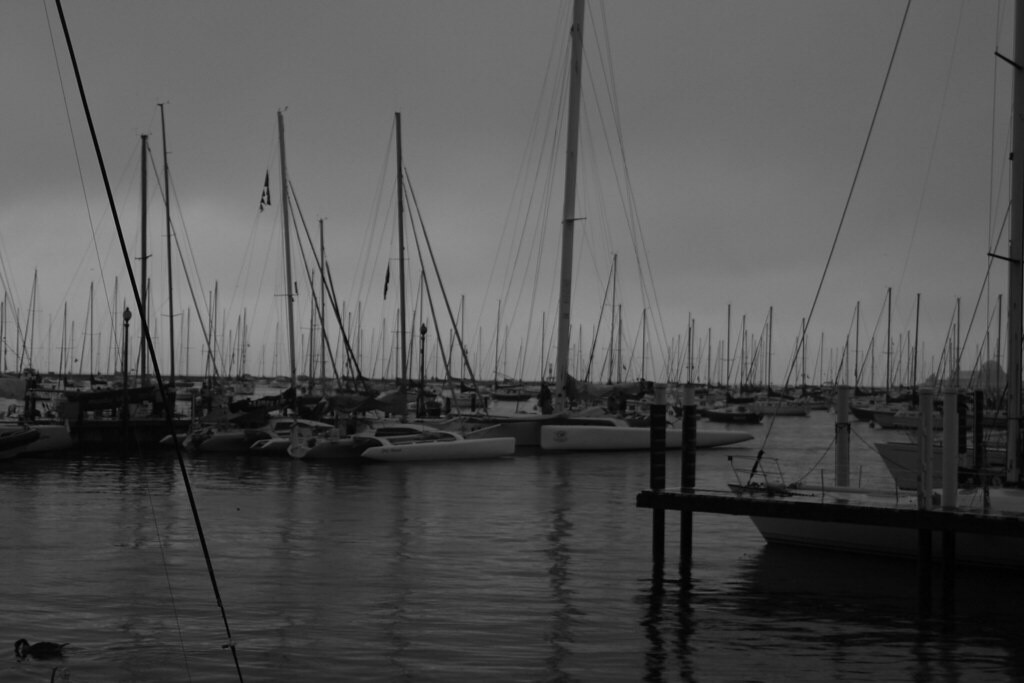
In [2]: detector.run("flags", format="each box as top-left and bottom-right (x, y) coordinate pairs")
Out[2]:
(260, 170), (271, 212)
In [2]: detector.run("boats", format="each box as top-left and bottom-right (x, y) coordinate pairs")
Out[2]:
(739, 539), (1024, 634)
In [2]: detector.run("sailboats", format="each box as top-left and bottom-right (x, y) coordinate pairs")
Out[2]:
(0, 2), (1023, 558)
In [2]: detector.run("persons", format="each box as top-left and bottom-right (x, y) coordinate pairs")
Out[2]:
(607, 391), (627, 418)
(537, 384), (552, 415)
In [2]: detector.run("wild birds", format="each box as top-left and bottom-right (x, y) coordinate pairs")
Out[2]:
(15, 638), (70, 655)
